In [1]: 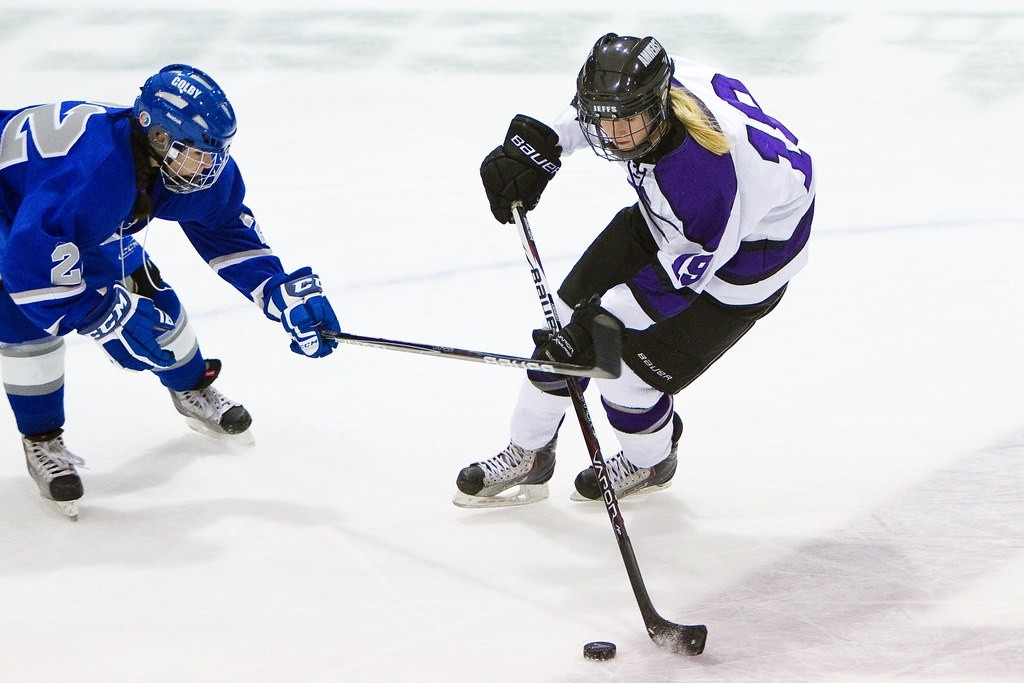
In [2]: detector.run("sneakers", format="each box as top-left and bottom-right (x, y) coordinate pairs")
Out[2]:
(167, 359), (256, 450)
(21, 427), (90, 522)
(452, 413), (566, 509)
(570, 411), (684, 502)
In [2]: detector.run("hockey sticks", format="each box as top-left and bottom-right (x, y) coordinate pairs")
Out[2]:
(319, 313), (622, 381)
(510, 200), (708, 658)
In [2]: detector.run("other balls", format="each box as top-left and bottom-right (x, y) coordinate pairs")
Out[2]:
(583, 641), (617, 662)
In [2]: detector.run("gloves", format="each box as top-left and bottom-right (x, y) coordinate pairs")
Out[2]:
(479, 114), (563, 225)
(73, 279), (177, 372)
(526, 302), (625, 397)
(260, 266), (341, 358)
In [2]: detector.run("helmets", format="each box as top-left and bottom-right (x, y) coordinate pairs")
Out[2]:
(570, 33), (675, 162)
(130, 64), (238, 195)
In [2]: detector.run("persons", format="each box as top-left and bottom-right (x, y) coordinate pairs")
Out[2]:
(0, 64), (341, 522)
(452, 33), (816, 508)
